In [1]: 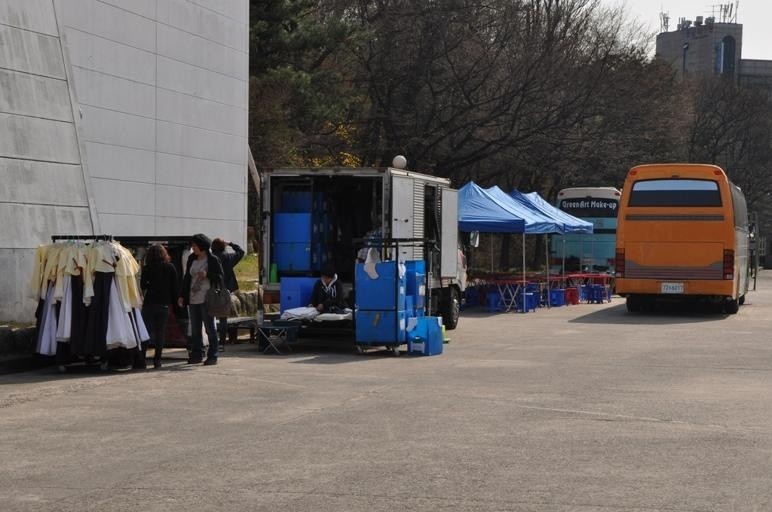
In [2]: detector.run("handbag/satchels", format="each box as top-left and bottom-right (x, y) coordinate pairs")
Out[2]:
(206, 275), (238, 318)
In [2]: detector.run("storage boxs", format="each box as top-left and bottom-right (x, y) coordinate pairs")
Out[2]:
(272, 190), (443, 355)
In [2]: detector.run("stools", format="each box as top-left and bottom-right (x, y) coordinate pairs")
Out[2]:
(483, 292), (504, 312)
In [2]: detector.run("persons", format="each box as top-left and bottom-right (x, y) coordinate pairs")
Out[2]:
(131, 243), (178, 372)
(308, 262), (346, 315)
(209, 237), (247, 346)
(179, 232), (226, 368)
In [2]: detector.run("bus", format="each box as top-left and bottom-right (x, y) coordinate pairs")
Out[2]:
(549, 187), (627, 298)
(613, 162), (753, 315)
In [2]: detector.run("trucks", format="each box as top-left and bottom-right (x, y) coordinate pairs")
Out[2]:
(259, 162), (480, 353)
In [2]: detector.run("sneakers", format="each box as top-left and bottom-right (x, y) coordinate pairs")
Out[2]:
(188, 356), (202, 363)
(204, 358), (217, 365)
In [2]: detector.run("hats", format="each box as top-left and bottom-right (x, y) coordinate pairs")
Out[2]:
(320, 263), (336, 277)
